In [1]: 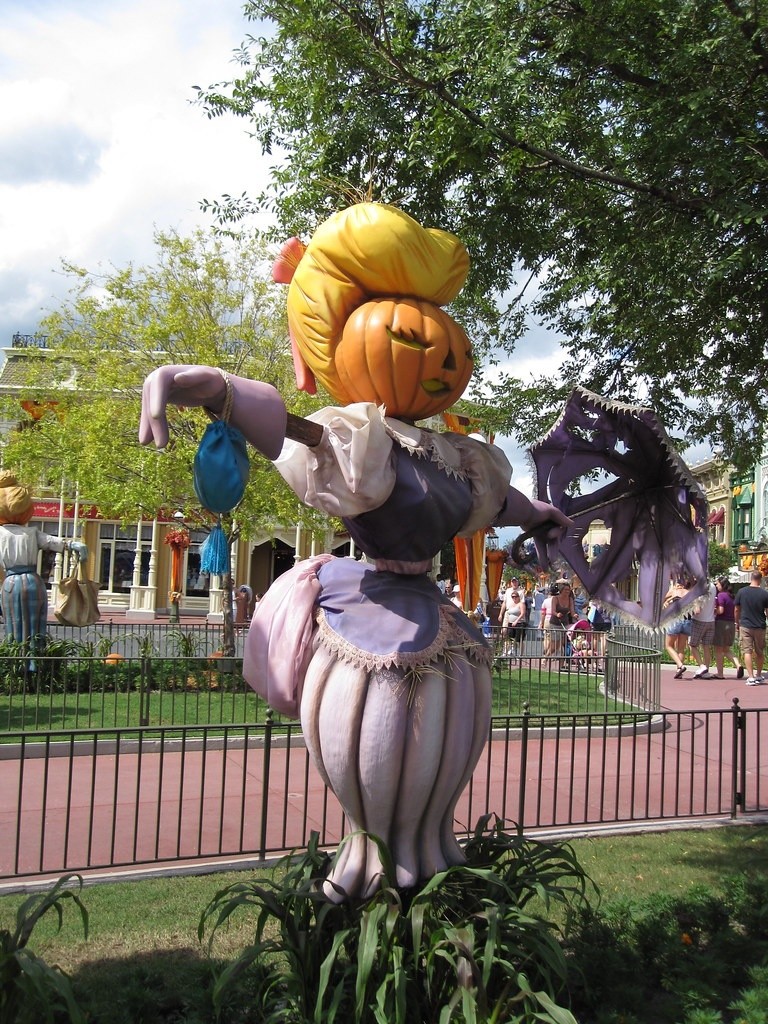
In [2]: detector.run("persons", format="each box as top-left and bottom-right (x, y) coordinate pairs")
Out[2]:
(0, 470), (88, 674)
(468, 577), (602, 674)
(435, 573), (462, 609)
(254, 592), (263, 610)
(665, 571), (768, 686)
(231, 578), (248, 635)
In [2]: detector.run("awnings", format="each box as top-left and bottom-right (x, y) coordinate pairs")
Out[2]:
(730, 487), (752, 510)
(705, 508), (724, 526)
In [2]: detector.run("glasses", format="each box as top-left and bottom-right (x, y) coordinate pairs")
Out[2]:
(512, 595), (518, 598)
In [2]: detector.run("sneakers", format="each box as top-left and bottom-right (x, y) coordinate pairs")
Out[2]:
(755, 676), (764, 684)
(745, 678), (755, 685)
(693, 665), (708, 679)
(702, 673), (710, 678)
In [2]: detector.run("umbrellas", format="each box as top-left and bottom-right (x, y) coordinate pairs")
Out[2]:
(511, 385), (708, 628)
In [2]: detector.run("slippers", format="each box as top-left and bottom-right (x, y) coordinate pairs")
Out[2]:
(737, 666), (744, 678)
(711, 673), (725, 679)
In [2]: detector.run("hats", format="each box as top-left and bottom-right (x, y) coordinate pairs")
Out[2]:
(451, 584), (460, 593)
(511, 576), (519, 582)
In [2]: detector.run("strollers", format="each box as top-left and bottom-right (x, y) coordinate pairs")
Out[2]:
(556, 613), (605, 674)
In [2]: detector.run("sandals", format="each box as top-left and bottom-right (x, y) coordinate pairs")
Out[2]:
(674, 664), (686, 678)
